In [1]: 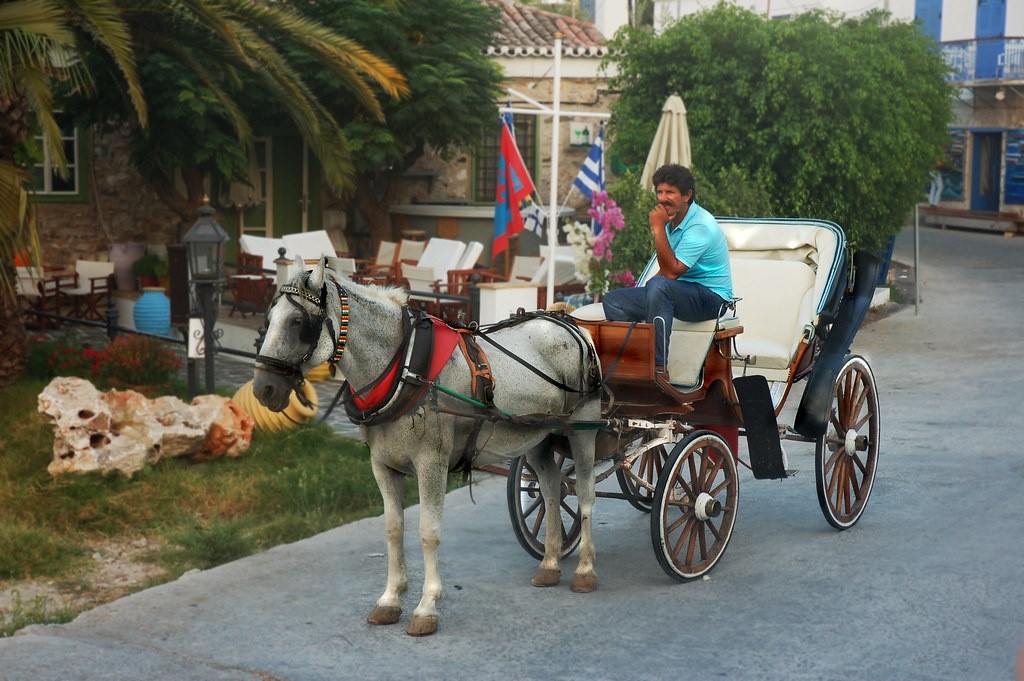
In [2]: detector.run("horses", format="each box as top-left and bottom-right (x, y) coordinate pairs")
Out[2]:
(252, 253), (603, 636)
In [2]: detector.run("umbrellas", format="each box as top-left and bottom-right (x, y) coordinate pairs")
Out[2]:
(640, 90), (692, 191)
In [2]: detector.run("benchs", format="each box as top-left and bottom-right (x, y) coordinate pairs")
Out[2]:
(920, 206), (1017, 232)
(653, 259), (820, 369)
(568, 303), (747, 381)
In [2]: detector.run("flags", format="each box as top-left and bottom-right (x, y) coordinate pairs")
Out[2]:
(572, 123), (608, 239)
(490, 100), (546, 259)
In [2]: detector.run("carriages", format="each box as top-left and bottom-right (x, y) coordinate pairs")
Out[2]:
(244, 215), (881, 636)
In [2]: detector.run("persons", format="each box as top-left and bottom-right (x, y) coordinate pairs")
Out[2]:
(604, 166), (734, 379)
(928, 159), (945, 210)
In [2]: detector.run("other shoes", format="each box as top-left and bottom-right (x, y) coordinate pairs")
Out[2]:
(657, 369), (669, 382)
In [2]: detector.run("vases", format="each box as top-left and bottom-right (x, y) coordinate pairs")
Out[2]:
(105, 376), (169, 393)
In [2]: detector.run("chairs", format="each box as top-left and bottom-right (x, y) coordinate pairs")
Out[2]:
(18, 231), (545, 336)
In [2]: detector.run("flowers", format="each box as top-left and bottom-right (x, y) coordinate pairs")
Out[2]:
(83, 332), (185, 391)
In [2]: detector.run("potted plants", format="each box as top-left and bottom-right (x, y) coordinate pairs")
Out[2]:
(134, 252), (171, 295)
